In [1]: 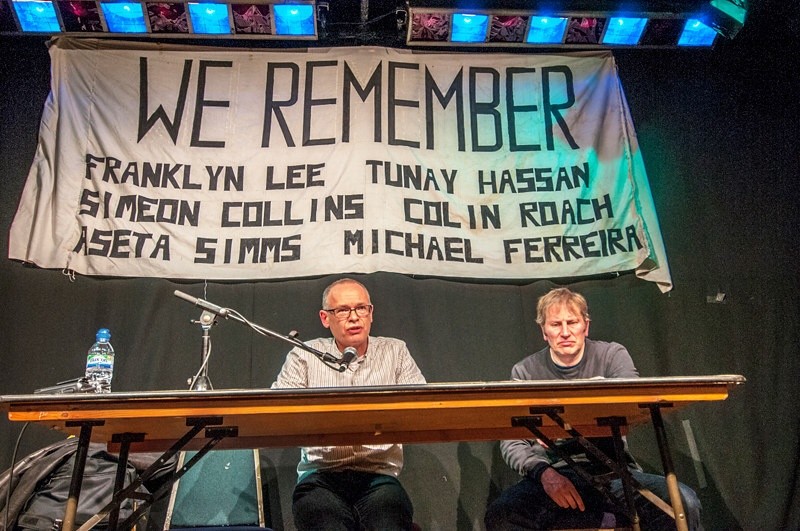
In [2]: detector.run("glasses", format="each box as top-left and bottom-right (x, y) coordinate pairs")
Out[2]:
(327, 305), (371, 320)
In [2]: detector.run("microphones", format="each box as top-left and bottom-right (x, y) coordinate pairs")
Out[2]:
(339, 347), (356, 372)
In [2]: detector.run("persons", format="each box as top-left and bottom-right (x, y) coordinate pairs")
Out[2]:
(485, 288), (702, 531)
(270, 279), (427, 531)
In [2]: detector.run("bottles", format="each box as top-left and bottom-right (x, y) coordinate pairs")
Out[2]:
(85, 328), (115, 393)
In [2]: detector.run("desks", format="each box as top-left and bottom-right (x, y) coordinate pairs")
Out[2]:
(0, 372), (750, 531)
